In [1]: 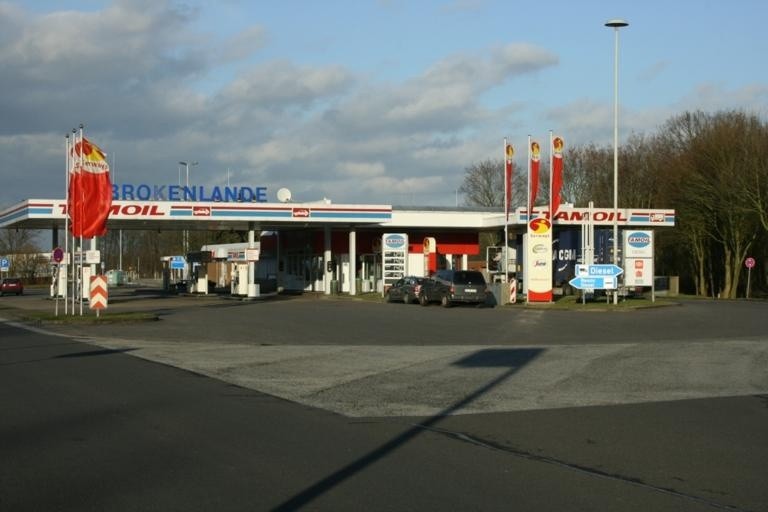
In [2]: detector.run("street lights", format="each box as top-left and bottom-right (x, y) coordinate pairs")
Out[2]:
(179, 159), (199, 259)
(604, 20), (629, 308)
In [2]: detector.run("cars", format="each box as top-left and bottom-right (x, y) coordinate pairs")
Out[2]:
(384, 277), (452, 306)
(427, 270), (488, 308)
(0, 278), (23, 297)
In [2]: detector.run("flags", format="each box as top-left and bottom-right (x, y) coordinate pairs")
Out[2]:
(551, 135), (564, 216)
(66, 134), (114, 239)
(529, 139), (541, 216)
(505, 144), (514, 222)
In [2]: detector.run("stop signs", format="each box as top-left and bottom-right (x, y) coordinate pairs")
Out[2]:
(54, 248), (63, 262)
(746, 258), (756, 268)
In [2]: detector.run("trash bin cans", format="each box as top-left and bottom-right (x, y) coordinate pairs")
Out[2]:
(330, 280), (338, 293)
(107, 271), (120, 287)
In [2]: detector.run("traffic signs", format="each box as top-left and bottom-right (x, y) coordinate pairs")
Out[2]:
(574, 264), (623, 278)
(568, 277), (618, 290)
(171, 261), (184, 268)
(172, 256), (184, 261)
